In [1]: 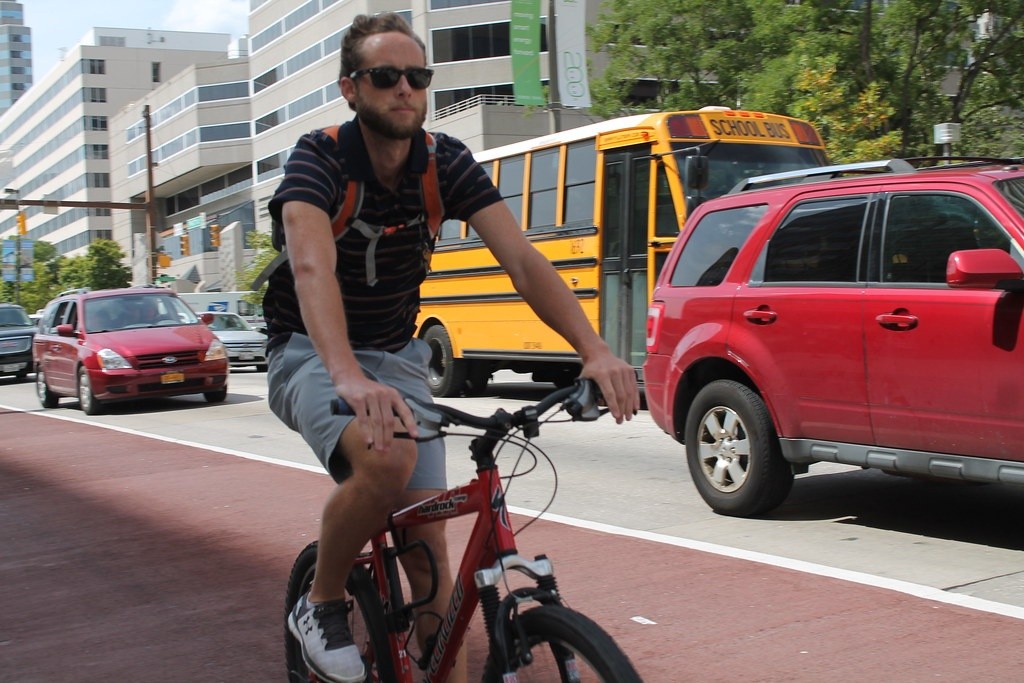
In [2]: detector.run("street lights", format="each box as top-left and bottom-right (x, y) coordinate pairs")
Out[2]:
(934, 123), (962, 165)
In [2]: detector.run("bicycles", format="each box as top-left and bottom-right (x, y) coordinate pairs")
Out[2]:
(285, 382), (644, 683)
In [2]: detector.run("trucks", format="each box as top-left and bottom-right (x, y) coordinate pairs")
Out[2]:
(157, 291), (268, 334)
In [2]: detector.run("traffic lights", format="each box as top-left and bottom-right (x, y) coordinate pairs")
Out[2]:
(180, 234), (190, 257)
(16, 212), (26, 237)
(210, 225), (221, 248)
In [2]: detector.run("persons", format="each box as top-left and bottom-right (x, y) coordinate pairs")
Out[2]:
(85, 300), (159, 330)
(262, 11), (639, 683)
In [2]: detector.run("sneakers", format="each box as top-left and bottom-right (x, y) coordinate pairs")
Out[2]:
(288, 581), (368, 683)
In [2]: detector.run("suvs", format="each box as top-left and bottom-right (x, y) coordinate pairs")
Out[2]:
(641, 156), (1024, 517)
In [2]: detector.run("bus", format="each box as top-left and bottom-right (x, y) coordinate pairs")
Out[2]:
(414, 103), (831, 398)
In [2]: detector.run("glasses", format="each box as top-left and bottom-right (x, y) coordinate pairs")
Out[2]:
(349, 65), (435, 90)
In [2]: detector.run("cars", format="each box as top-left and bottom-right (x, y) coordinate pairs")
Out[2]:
(32, 286), (230, 416)
(0, 302), (39, 381)
(177, 312), (268, 372)
(30, 310), (65, 334)
